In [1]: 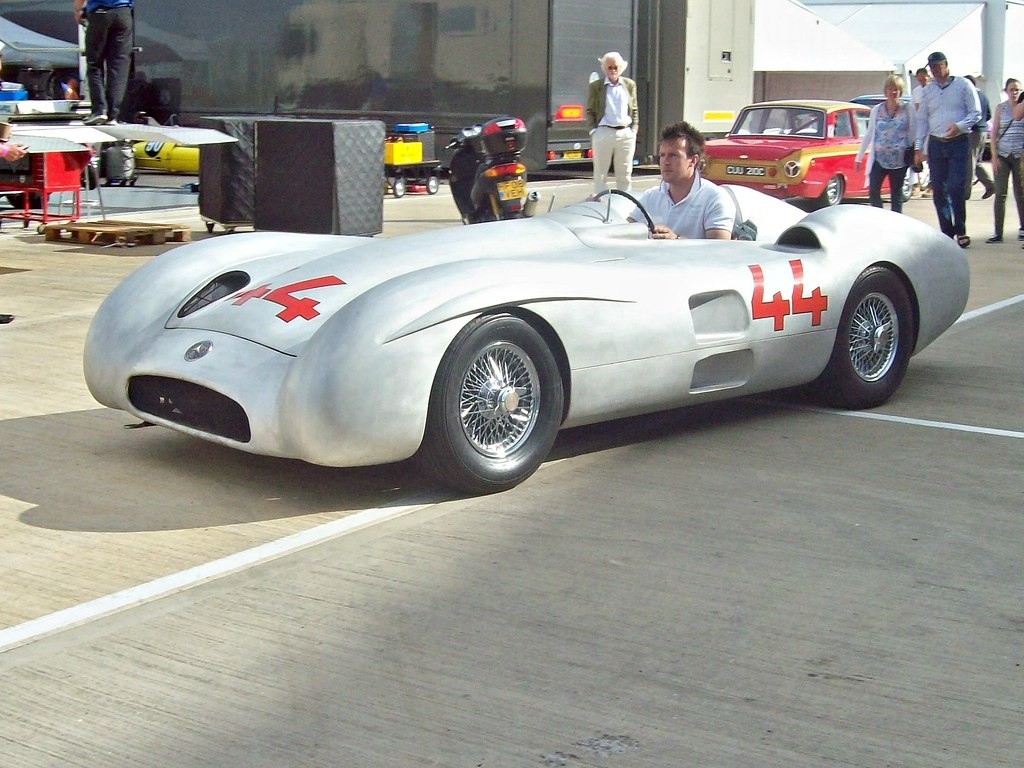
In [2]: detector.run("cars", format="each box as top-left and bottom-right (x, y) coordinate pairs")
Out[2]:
(701, 93), (927, 206)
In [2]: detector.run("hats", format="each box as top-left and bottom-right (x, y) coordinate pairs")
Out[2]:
(926, 52), (947, 66)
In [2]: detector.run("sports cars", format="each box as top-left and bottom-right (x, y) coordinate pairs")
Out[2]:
(82, 185), (977, 498)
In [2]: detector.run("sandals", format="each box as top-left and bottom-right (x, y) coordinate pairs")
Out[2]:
(956, 234), (971, 249)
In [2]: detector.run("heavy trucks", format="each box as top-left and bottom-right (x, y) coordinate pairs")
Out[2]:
(0, 0), (753, 174)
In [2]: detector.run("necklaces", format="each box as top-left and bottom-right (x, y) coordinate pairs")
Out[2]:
(885, 103), (900, 119)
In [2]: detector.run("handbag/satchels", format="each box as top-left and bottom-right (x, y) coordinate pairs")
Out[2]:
(904, 146), (924, 173)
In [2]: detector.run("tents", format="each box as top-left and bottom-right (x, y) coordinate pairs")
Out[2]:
(0, 13), (78, 67)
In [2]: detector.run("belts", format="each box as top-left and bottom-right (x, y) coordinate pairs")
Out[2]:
(603, 125), (627, 130)
(930, 135), (964, 143)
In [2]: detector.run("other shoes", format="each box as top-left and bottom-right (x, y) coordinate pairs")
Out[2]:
(100, 117), (119, 126)
(1017, 228), (1024, 242)
(921, 188), (933, 198)
(986, 234), (1006, 243)
(82, 111), (109, 126)
(982, 187), (997, 199)
(910, 183), (922, 197)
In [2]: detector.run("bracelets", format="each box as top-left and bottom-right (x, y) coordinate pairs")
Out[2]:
(676, 235), (684, 239)
(0, 146), (8, 157)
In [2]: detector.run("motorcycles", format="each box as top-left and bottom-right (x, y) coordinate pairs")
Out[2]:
(443, 116), (538, 225)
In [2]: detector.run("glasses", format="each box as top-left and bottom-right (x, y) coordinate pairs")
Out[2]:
(607, 65), (618, 71)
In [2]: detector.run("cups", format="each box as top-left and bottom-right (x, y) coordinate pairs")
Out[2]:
(0, 122), (13, 142)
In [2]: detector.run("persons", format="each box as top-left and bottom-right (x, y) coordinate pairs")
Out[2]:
(912, 69), (934, 197)
(0, 54), (28, 324)
(586, 120), (737, 240)
(963, 74), (995, 199)
(986, 77), (1024, 243)
(586, 52), (639, 193)
(1013, 91), (1024, 121)
(915, 52), (983, 246)
(854, 75), (917, 213)
(73, 0), (134, 126)
